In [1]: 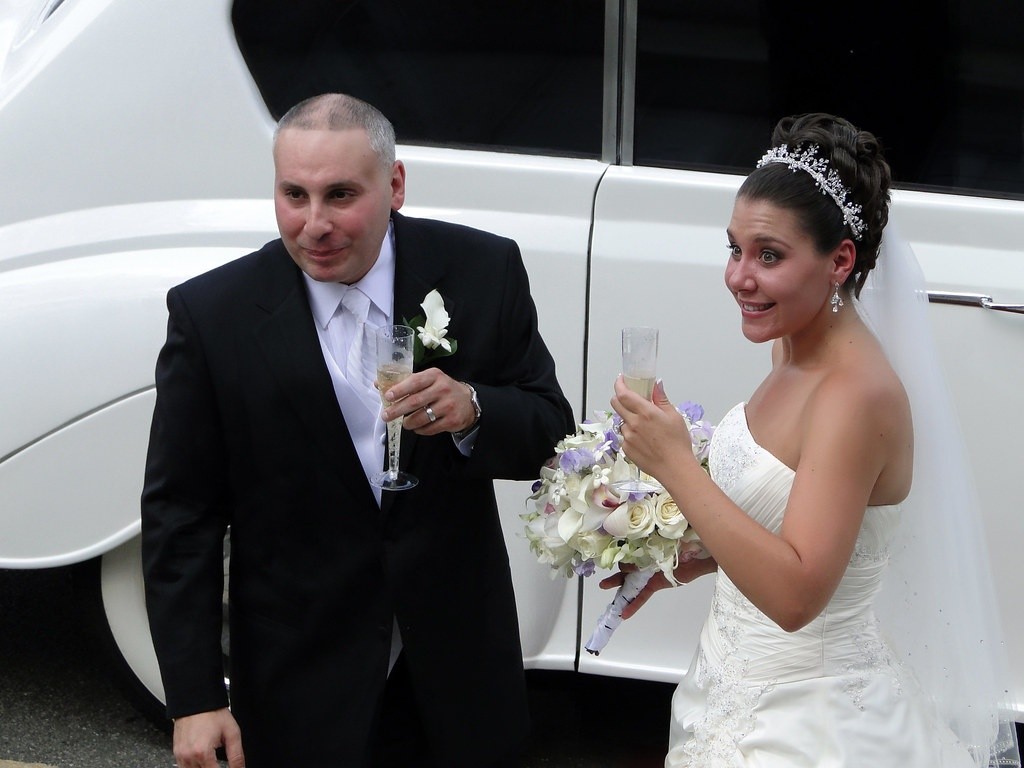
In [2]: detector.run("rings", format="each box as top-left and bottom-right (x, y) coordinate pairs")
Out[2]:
(423, 404), (436, 423)
(618, 419), (625, 433)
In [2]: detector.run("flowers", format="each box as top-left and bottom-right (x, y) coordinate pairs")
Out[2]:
(519, 399), (715, 656)
(403, 288), (457, 364)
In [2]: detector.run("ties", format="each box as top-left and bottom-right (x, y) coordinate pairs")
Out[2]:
(345, 289), (382, 397)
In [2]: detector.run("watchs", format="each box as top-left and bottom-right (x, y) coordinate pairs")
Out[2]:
(452, 382), (482, 439)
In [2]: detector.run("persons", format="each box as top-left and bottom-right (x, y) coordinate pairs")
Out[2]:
(140, 92), (578, 768)
(599, 113), (980, 768)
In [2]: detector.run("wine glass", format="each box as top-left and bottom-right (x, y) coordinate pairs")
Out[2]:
(369, 325), (418, 491)
(608, 328), (662, 493)
